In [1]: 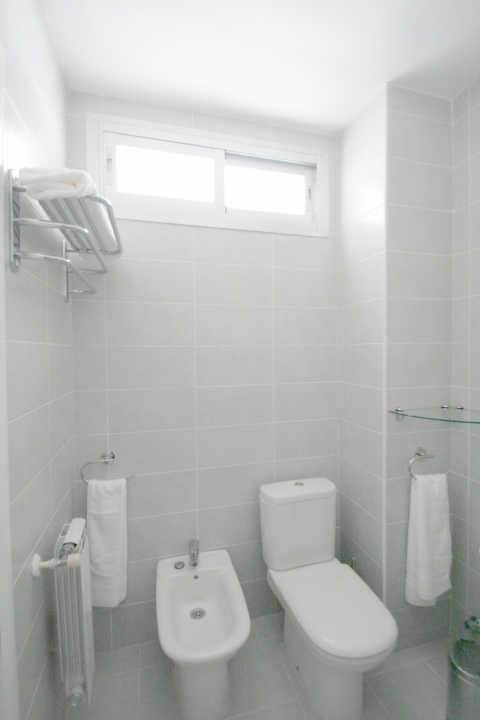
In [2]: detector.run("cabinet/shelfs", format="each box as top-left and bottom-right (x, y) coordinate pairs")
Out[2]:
(8, 168), (122, 302)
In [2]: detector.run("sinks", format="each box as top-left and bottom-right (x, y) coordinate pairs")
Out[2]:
(155, 548), (252, 667)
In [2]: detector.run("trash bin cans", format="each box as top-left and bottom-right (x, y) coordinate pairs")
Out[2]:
(446, 637), (480, 720)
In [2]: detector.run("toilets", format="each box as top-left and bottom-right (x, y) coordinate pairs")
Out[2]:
(258, 475), (398, 718)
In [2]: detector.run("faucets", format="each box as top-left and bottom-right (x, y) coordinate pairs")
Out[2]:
(186, 538), (200, 565)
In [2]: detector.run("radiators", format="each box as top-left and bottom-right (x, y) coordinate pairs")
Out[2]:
(51, 516), (97, 708)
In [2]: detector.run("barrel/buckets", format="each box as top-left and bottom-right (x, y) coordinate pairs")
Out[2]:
(448, 646), (479, 719)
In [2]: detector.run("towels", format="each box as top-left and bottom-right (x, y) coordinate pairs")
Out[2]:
(20, 170), (96, 202)
(86, 477), (128, 609)
(403, 474), (453, 608)
